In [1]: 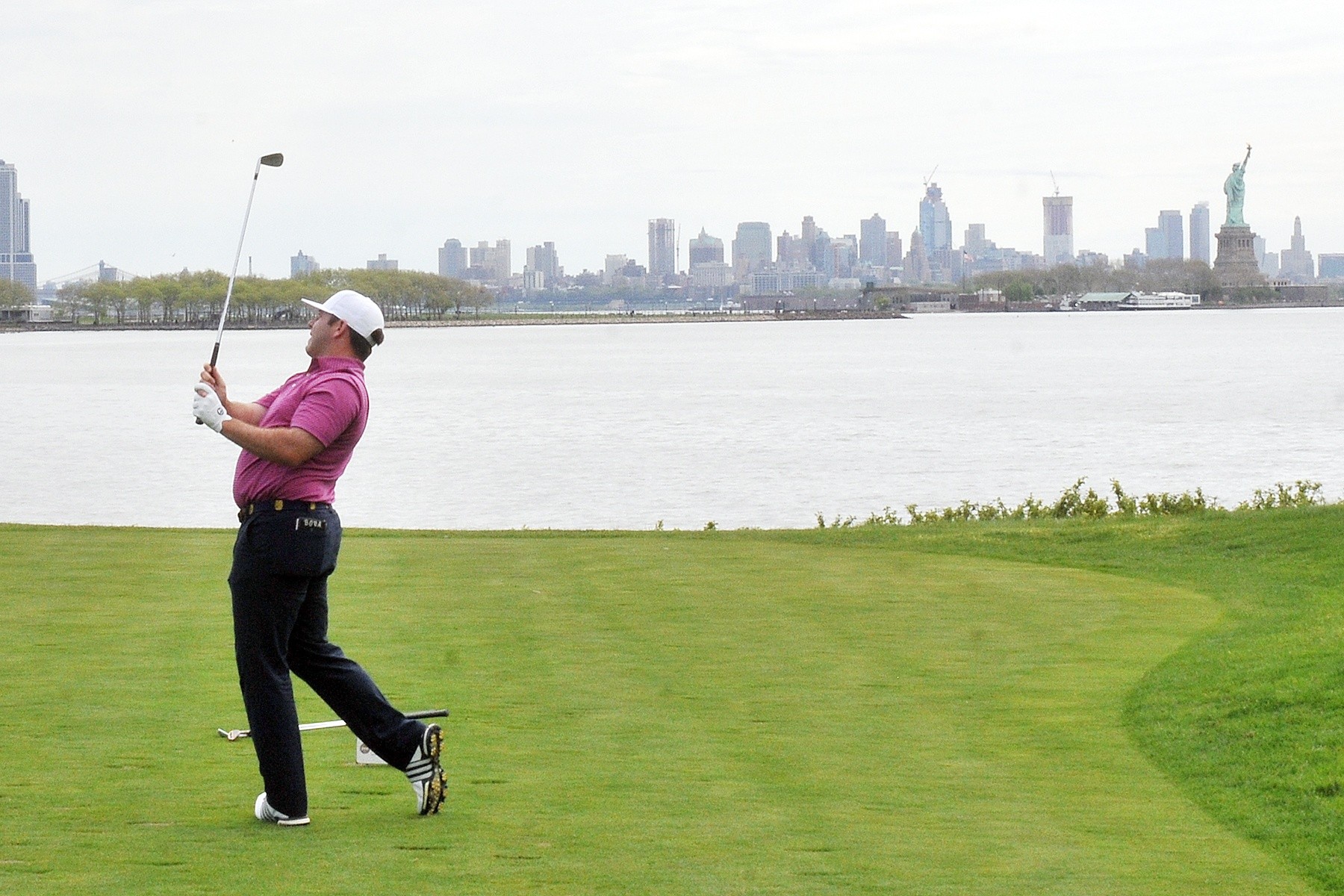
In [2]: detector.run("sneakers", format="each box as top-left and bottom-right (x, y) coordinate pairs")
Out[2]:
(403, 723), (447, 815)
(254, 792), (310, 825)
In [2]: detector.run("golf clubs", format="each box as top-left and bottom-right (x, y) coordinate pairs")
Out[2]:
(217, 709), (450, 740)
(195, 152), (284, 425)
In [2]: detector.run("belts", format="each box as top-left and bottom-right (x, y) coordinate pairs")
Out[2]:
(238, 500), (328, 522)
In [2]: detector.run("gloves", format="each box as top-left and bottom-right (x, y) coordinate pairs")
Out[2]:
(193, 382), (232, 432)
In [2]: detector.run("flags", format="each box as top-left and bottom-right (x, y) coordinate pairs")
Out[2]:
(964, 251), (973, 263)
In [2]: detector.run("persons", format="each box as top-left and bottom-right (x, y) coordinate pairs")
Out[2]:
(1223, 150), (1251, 224)
(192, 290), (448, 826)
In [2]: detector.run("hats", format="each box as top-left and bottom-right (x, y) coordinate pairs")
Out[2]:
(301, 290), (384, 346)
